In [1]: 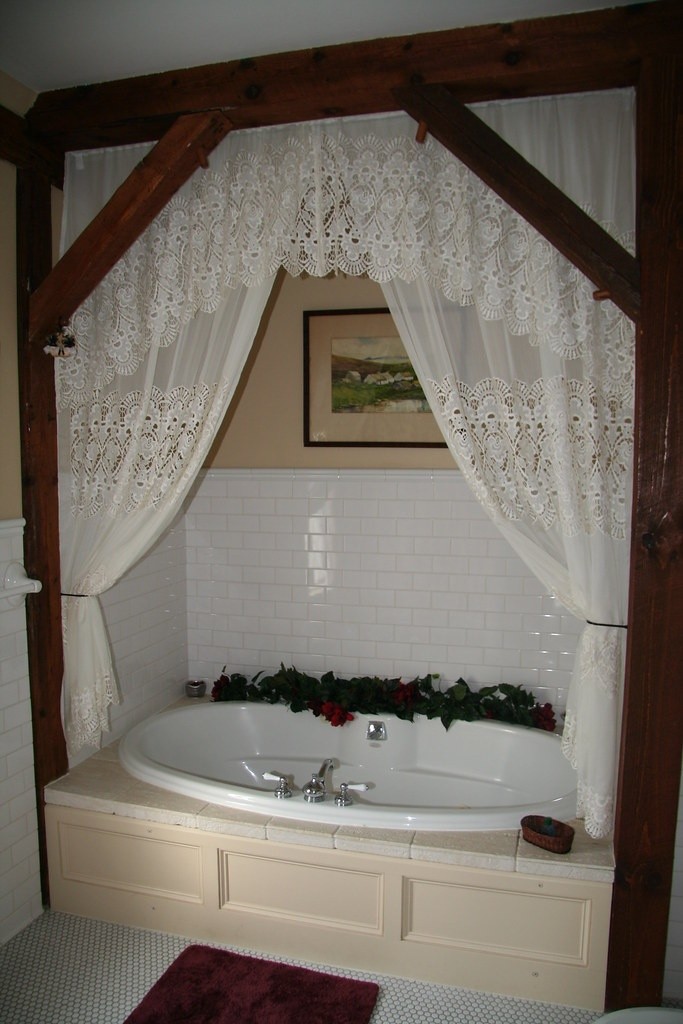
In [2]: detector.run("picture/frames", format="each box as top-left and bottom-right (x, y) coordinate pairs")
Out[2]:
(303, 307), (447, 448)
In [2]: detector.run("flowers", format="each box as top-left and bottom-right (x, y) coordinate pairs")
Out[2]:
(211, 662), (557, 732)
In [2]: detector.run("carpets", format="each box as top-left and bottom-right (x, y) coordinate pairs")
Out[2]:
(123, 945), (380, 1024)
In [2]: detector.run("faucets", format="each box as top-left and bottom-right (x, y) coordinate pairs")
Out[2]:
(302, 759), (335, 803)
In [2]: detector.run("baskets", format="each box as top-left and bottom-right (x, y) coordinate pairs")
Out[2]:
(521, 815), (575, 853)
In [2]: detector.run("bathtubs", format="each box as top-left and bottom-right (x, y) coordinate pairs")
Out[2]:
(118, 702), (577, 832)
